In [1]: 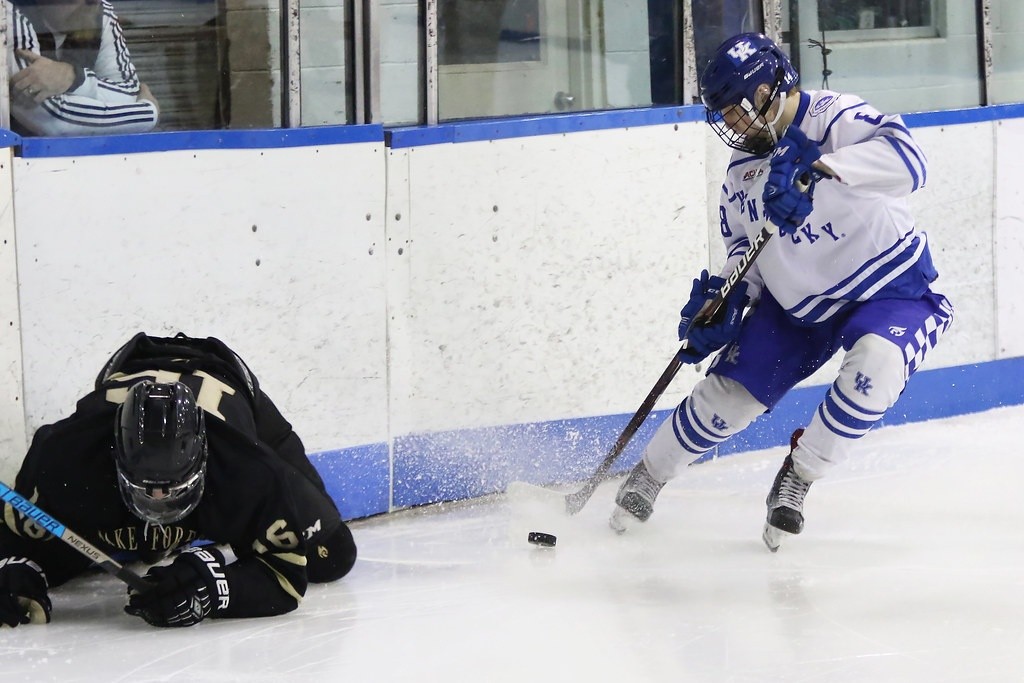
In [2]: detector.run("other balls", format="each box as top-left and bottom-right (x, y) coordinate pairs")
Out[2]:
(528, 531), (557, 548)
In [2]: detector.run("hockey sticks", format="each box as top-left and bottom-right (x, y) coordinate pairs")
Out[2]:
(506, 179), (810, 517)
(0, 480), (156, 595)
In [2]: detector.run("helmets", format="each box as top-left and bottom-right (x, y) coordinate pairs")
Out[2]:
(698, 31), (799, 156)
(113, 379), (208, 525)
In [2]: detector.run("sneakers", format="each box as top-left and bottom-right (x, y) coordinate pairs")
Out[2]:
(762, 427), (813, 552)
(610, 459), (668, 535)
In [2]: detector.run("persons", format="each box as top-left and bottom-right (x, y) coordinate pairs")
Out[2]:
(7, 0), (159, 136)
(0, 331), (358, 628)
(609, 30), (953, 552)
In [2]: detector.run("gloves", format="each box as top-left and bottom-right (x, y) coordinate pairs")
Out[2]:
(0, 564), (51, 628)
(677, 269), (750, 357)
(122, 559), (210, 628)
(763, 125), (822, 234)
